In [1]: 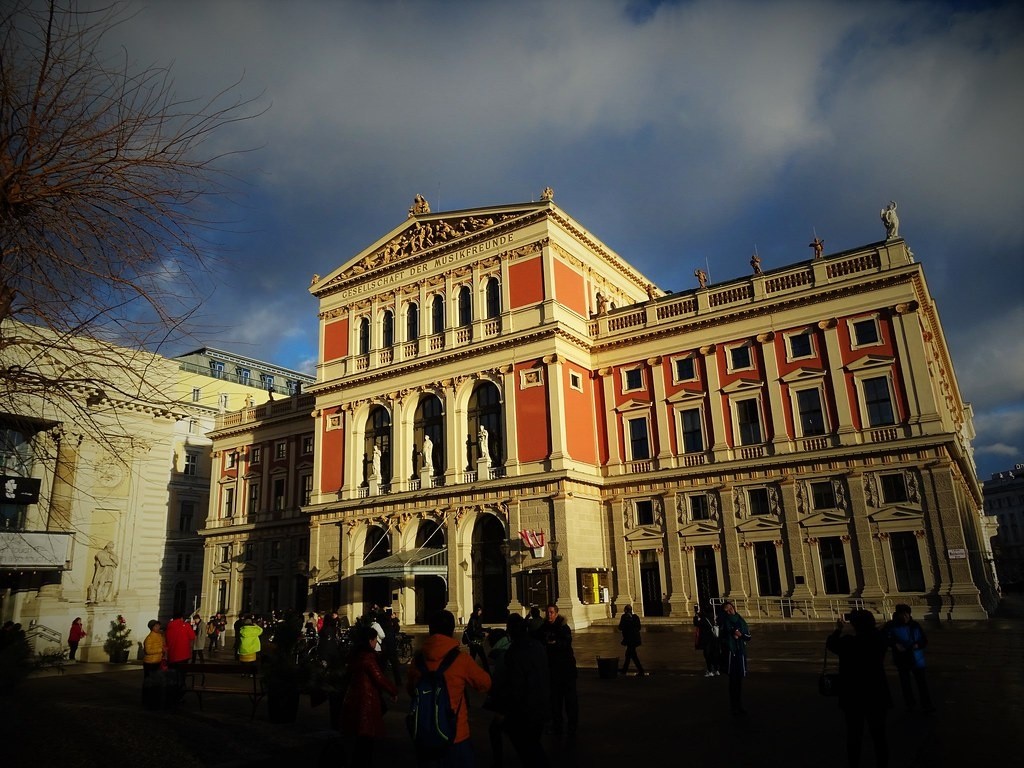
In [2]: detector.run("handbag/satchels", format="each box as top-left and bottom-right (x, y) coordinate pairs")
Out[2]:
(711, 625), (719, 637)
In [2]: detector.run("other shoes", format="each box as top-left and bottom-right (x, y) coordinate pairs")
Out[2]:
(200, 660), (205, 664)
(635, 672), (644, 676)
(220, 646), (225, 651)
(545, 729), (563, 736)
(617, 672), (626, 677)
(187, 662), (195, 664)
(564, 732), (574, 738)
(488, 763), (503, 768)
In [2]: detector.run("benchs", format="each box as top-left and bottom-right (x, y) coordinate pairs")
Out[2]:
(179, 662), (270, 722)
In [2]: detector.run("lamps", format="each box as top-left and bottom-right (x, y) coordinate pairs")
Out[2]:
(298, 556), (344, 588)
(501, 538), (562, 562)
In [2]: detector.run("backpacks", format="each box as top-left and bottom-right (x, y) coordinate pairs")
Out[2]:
(404, 646), (457, 751)
(316, 734), (345, 768)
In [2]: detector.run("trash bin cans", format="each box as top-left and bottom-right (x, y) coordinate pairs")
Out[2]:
(263, 673), (300, 724)
(596, 654), (620, 681)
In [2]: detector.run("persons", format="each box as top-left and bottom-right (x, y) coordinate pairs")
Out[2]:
(596, 292), (609, 315)
(826, 610), (895, 768)
(372, 446), (381, 474)
(295, 379), (303, 394)
(89, 541), (119, 602)
(618, 605), (643, 678)
(646, 284), (656, 300)
(408, 192), (430, 217)
(311, 274), (319, 284)
(142, 621), (168, 705)
(382, 217), (493, 263)
(694, 270), (708, 287)
(165, 604), (400, 686)
(406, 606), (576, 768)
(478, 424), (489, 458)
(68, 618), (86, 661)
(342, 626), (398, 768)
(0, 620), (25, 673)
(881, 604), (936, 716)
(693, 599), (752, 719)
(218, 392), (225, 414)
(245, 393), (253, 406)
(750, 254), (761, 273)
(540, 186), (554, 199)
(420, 435), (433, 467)
(809, 237), (824, 258)
(880, 201), (899, 237)
(267, 387), (275, 400)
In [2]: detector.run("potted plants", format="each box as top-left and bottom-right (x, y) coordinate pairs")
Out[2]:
(104, 613), (131, 664)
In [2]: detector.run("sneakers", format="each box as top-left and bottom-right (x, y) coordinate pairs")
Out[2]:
(704, 671), (714, 677)
(714, 671), (720, 675)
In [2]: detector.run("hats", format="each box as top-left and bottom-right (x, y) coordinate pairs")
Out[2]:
(148, 620), (161, 630)
